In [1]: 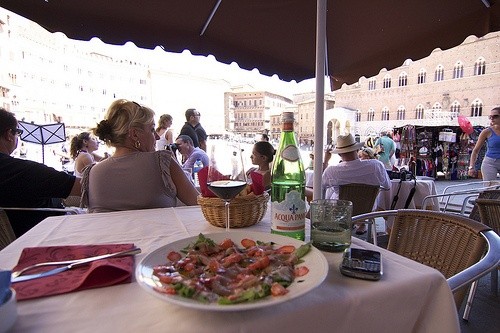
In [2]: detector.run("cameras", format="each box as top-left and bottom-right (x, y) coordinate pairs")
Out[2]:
(164, 143), (178, 151)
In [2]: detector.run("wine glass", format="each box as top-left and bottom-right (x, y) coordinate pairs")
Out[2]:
(206, 142), (247, 232)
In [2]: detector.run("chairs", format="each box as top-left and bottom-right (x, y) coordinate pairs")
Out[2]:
(350, 209), (500, 311)
(421, 180), (500, 236)
(0, 207), (72, 249)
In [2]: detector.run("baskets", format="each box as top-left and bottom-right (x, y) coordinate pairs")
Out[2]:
(197, 193), (270, 228)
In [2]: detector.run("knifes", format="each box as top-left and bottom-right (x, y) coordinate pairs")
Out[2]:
(11, 247), (141, 282)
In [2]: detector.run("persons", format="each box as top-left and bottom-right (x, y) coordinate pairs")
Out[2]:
(180, 109), (209, 154)
(246, 141), (277, 195)
(305, 153), (315, 171)
(355, 129), (405, 234)
(88, 100), (203, 212)
(318, 132), (393, 213)
(0, 108), (86, 239)
(155, 113), (173, 152)
(68, 131), (112, 188)
(468, 107), (500, 187)
(176, 135), (209, 177)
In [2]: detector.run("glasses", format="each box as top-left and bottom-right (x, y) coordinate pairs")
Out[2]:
(194, 112), (200, 117)
(12, 128), (23, 136)
(128, 101), (142, 128)
(490, 114), (500, 119)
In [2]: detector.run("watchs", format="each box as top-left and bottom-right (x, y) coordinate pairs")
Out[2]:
(468, 166), (475, 170)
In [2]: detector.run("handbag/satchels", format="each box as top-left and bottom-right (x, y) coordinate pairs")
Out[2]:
(377, 143), (385, 154)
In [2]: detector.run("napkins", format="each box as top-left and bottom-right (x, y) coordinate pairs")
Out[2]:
(11, 244), (135, 300)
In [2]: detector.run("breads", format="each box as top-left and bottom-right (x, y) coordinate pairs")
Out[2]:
(235, 185), (255, 198)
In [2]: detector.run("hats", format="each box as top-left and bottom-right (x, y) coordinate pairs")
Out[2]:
(329, 133), (365, 154)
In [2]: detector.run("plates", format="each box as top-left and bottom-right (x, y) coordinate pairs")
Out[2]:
(135, 232), (329, 311)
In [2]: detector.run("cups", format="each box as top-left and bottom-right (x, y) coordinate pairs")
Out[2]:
(310, 198), (352, 252)
(0, 287), (19, 333)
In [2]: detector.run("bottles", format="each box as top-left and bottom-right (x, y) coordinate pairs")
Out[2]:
(270, 112), (306, 242)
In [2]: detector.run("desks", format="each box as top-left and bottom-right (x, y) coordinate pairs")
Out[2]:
(0, 196), (460, 333)
(372, 178), (439, 211)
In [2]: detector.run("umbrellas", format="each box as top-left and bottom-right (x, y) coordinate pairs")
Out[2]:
(0, 0), (500, 201)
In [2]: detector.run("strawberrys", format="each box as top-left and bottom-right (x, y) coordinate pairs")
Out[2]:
(153, 238), (309, 299)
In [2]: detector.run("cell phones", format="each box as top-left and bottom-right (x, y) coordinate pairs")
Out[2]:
(339, 247), (382, 280)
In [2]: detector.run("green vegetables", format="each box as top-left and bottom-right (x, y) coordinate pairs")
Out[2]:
(173, 233), (311, 304)
